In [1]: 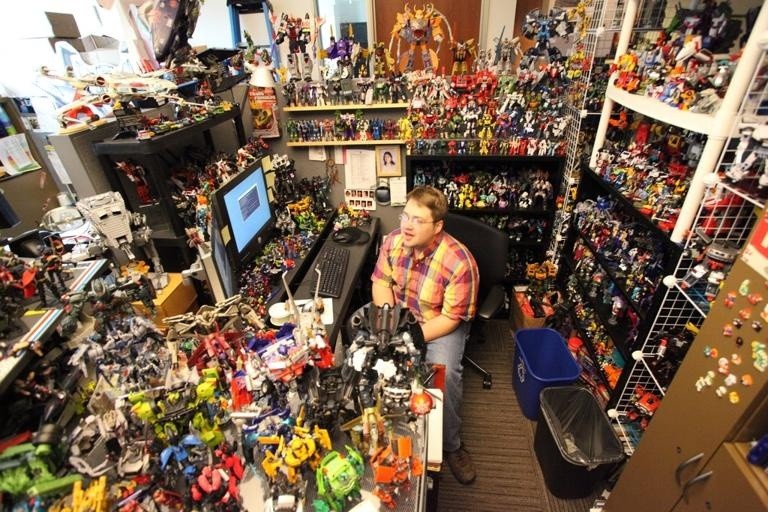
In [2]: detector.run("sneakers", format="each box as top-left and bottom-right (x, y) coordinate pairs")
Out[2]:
(445, 443), (477, 485)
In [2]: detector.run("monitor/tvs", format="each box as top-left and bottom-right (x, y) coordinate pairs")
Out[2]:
(211, 157), (278, 271)
(197, 196), (235, 303)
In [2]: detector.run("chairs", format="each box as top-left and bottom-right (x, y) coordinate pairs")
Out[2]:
(440, 213), (511, 389)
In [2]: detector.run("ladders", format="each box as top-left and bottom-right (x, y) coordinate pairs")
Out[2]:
(226, 0), (281, 82)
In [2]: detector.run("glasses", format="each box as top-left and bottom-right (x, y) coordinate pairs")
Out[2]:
(399, 212), (435, 226)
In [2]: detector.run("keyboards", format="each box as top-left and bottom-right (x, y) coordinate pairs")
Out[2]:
(310, 244), (350, 298)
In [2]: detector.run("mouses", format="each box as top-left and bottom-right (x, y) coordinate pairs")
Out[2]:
(301, 300), (325, 314)
(336, 231), (360, 244)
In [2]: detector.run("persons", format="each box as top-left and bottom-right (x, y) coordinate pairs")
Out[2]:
(0, 0), (768, 512)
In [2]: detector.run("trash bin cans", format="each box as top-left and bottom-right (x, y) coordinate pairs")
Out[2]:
(512, 327), (582, 422)
(533, 386), (625, 500)
